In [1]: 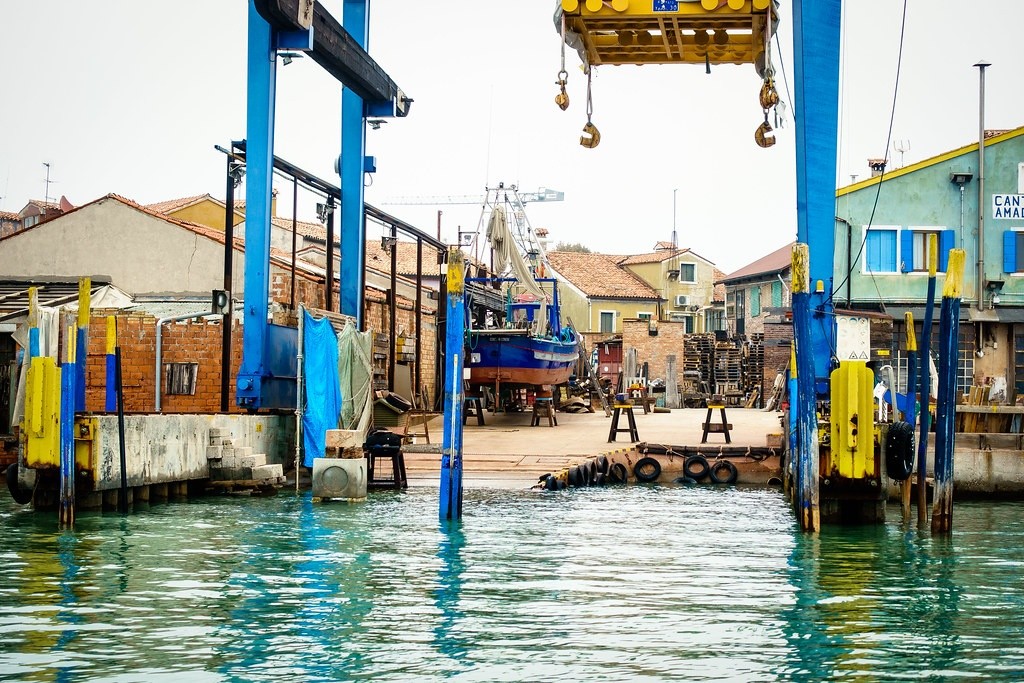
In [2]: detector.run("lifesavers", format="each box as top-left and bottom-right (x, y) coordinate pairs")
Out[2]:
(683, 456), (709, 480)
(633, 457), (662, 481)
(609, 462), (628, 484)
(671, 477), (698, 484)
(710, 460), (738, 485)
(886, 420), (915, 482)
(541, 454), (608, 491)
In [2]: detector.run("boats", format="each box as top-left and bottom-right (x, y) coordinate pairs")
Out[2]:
(460, 182), (584, 388)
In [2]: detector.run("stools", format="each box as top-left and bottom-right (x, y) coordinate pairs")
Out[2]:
(530, 397), (558, 428)
(701, 405), (732, 445)
(461, 397), (486, 427)
(403, 408), (430, 446)
(366, 450), (407, 490)
(607, 404), (639, 444)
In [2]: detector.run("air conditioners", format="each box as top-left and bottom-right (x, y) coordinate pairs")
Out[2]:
(675, 295), (691, 306)
